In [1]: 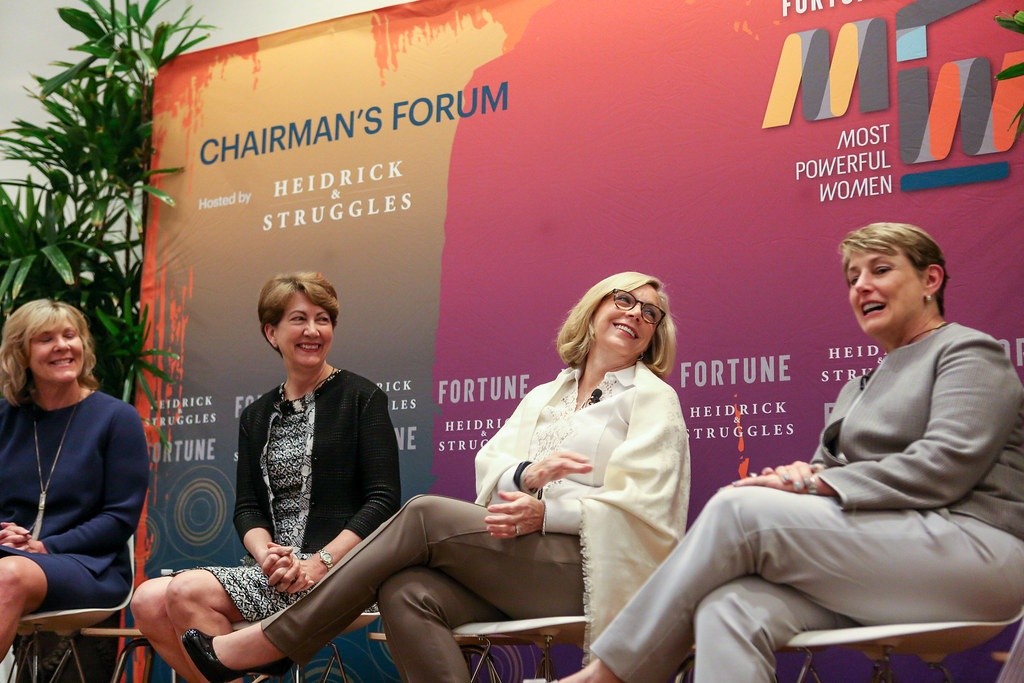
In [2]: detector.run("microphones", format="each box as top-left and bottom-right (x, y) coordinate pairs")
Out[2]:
(590, 389), (602, 404)
(280, 401), (289, 416)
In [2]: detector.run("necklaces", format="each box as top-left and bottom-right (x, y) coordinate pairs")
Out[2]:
(281, 366), (335, 423)
(27, 387), (82, 510)
(929, 321), (948, 334)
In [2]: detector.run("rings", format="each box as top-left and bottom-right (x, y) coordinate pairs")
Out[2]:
(515, 523), (520, 536)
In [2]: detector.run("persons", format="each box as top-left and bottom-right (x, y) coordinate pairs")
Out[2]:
(522, 223), (1023, 683)
(0, 299), (150, 666)
(130, 271), (693, 682)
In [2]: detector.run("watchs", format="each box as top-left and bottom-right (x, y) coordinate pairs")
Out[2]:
(318, 548), (334, 572)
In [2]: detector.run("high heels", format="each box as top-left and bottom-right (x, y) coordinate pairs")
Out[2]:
(181, 628), (294, 683)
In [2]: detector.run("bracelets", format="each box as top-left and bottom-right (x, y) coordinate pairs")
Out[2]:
(808, 475), (817, 495)
(542, 509), (546, 537)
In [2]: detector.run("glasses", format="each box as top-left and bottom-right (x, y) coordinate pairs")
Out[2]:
(605, 289), (666, 331)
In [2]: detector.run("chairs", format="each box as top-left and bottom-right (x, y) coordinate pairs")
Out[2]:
(7, 533), (1024, 683)
